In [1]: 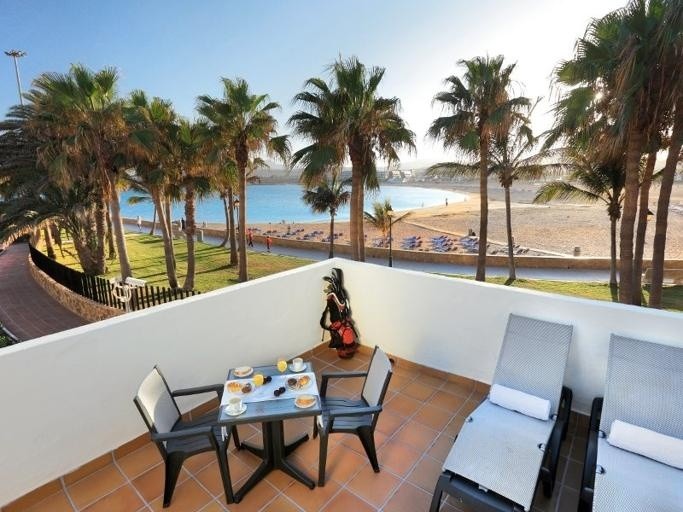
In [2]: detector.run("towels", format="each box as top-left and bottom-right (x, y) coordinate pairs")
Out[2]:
(488, 383), (552, 421)
(606, 419), (683, 472)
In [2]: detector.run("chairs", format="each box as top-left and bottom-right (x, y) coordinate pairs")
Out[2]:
(134, 365), (238, 508)
(428, 313), (572, 512)
(312, 345), (394, 486)
(576, 331), (683, 512)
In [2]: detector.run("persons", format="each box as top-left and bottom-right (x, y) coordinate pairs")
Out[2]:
(180, 217), (185, 231)
(137, 215), (141, 231)
(247, 228), (254, 247)
(267, 236), (272, 252)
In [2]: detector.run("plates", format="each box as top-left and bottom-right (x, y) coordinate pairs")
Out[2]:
(224, 403), (247, 416)
(294, 394), (316, 409)
(233, 367), (254, 377)
(288, 364), (307, 372)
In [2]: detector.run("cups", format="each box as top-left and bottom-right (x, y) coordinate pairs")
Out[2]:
(228, 396), (242, 412)
(293, 358), (304, 371)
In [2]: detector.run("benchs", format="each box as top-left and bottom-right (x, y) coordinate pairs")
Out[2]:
(641, 268), (683, 284)
